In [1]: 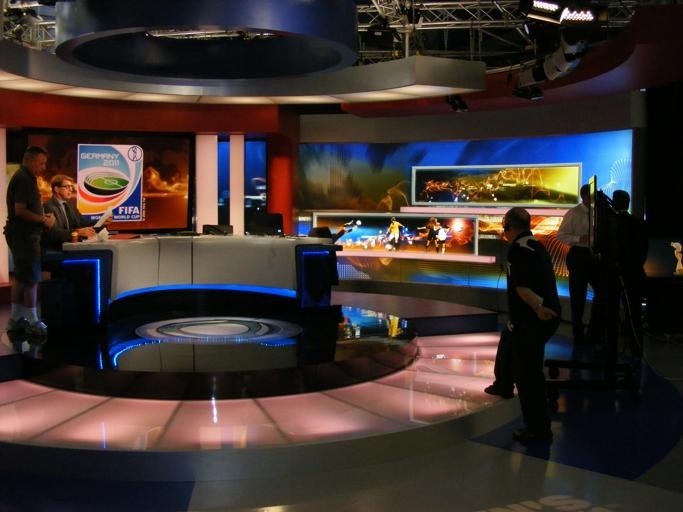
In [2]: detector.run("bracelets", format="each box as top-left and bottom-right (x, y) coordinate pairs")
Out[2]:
(533, 303), (542, 311)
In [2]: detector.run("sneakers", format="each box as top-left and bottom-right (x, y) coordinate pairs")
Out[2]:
(484, 382), (514, 399)
(512, 428), (553, 443)
(7, 316), (28, 333)
(24, 321), (48, 337)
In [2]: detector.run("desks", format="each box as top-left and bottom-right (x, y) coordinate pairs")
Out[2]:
(57, 234), (342, 324)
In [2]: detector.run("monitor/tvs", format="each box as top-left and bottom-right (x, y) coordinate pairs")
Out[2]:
(588, 176), (597, 250)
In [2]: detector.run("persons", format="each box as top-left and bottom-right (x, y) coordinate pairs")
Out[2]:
(43, 173), (113, 271)
(386, 216), (404, 250)
(435, 226), (449, 254)
(3, 144), (55, 336)
(344, 233), (384, 248)
(417, 222), (441, 253)
(330, 220), (358, 241)
(483, 207), (562, 444)
(557, 183), (595, 337)
(611, 187), (649, 360)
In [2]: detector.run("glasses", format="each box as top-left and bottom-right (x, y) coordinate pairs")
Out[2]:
(58, 184), (73, 190)
